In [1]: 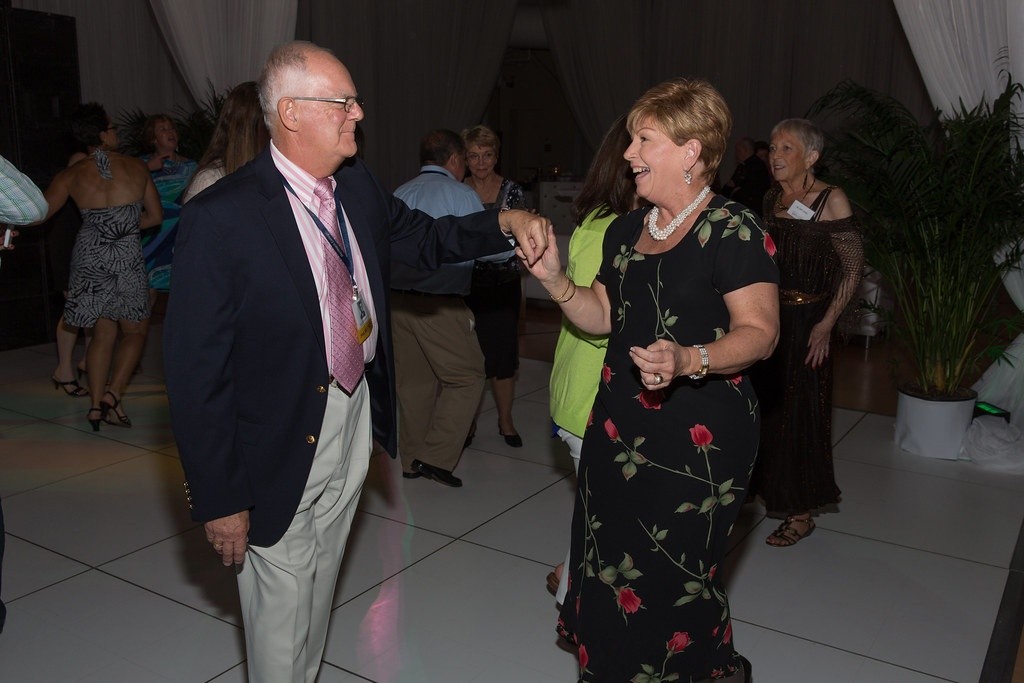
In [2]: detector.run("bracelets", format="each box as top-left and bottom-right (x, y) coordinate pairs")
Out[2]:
(561, 279), (577, 303)
(549, 274), (569, 300)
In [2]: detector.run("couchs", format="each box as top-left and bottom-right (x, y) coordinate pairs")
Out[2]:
(837, 229), (902, 350)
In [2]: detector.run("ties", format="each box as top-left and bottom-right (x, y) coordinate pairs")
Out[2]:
(312, 176), (366, 393)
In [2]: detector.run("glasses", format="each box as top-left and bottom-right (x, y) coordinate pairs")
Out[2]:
(288, 94), (369, 112)
(104, 124), (119, 134)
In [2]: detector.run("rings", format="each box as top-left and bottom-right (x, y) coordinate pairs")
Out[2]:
(654, 374), (663, 384)
(214, 542), (223, 549)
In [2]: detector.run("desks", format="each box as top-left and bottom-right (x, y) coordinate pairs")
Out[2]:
(521, 235), (571, 302)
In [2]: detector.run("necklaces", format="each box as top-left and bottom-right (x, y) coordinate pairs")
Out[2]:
(647, 186), (712, 239)
(776, 177), (815, 209)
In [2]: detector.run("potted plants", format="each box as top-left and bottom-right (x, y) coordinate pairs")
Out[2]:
(803, 44), (1024, 461)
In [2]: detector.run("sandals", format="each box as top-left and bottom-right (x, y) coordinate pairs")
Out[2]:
(766, 515), (816, 546)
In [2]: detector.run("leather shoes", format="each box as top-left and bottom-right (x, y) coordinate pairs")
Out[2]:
(410, 459), (463, 488)
(402, 469), (422, 479)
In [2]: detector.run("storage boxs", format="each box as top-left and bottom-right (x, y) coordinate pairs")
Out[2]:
(538, 180), (584, 232)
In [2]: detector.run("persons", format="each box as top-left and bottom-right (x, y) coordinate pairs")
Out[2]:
(510, 74), (780, 683)
(385, 126), (519, 487)
(751, 118), (865, 540)
(545, 111), (646, 607)
(720, 135), (774, 207)
(158, 40), (550, 682)
(0, 156), (51, 250)
(183, 80), (270, 205)
(130, 112), (199, 375)
(29, 100), (163, 437)
(457, 127), (527, 447)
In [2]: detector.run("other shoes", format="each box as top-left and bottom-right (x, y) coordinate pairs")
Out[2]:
(546, 572), (560, 592)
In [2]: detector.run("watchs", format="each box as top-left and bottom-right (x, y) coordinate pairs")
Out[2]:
(688, 344), (709, 379)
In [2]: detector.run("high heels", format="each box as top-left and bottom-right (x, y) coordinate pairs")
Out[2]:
(75, 366), (111, 386)
(52, 375), (90, 398)
(463, 425), (477, 448)
(86, 408), (103, 430)
(497, 422), (524, 448)
(99, 391), (132, 427)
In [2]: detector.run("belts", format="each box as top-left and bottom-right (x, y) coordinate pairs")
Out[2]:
(777, 287), (821, 306)
(328, 363), (371, 383)
(393, 288), (461, 298)
